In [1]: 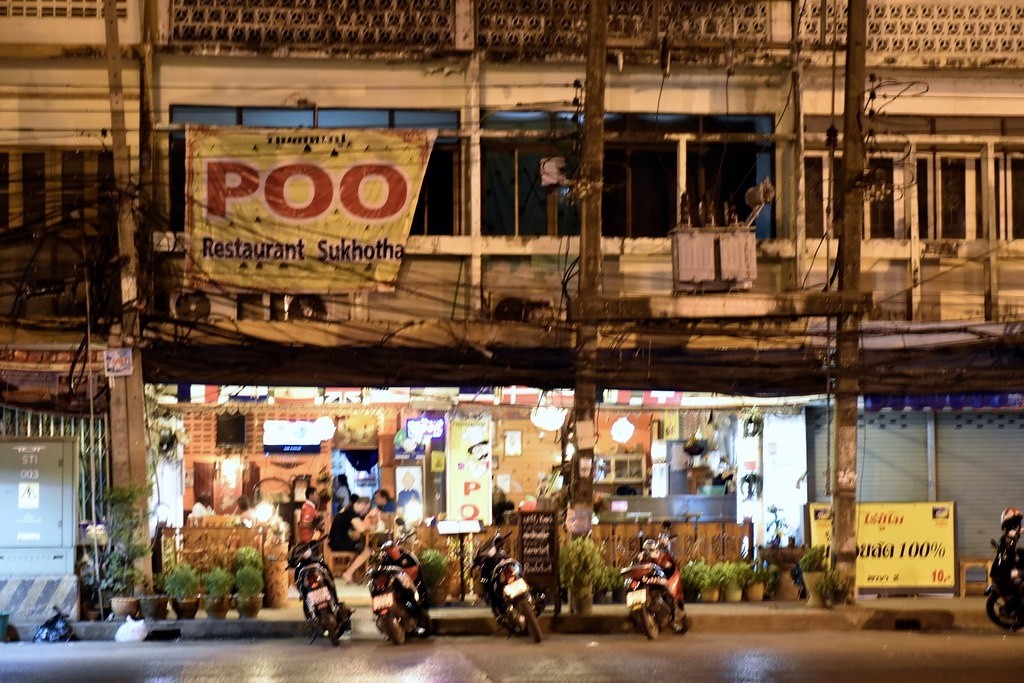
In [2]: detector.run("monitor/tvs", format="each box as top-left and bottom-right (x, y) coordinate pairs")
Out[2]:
(263, 419), (321, 452)
(406, 417), (444, 438)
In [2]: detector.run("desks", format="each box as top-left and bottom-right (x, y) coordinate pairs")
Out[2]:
(362, 529), (391, 575)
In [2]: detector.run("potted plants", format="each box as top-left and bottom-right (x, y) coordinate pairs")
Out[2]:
(163, 561), (202, 619)
(560, 536), (625, 615)
(233, 544), (266, 617)
(679, 556), (778, 603)
(202, 566), (238, 618)
(97, 477), (152, 618)
(418, 549), (451, 607)
(796, 545), (855, 609)
(138, 570), (168, 620)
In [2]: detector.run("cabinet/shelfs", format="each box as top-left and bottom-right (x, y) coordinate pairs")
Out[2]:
(594, 452), (648, 498)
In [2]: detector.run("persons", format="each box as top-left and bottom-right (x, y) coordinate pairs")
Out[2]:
(334, 474), (349, 512)
(493, 490), (514, 526)
(300, 487), (320, 542)
(234, 496), (254, 517)
(375, 490), (394, 511)
(329, 497), (378, 585)
(192, 496), (212, 527)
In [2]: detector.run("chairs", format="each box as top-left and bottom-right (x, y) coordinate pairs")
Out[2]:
(322, 512), (358, 581)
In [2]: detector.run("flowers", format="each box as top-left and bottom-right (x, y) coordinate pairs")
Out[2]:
(765, 504), (795, 549)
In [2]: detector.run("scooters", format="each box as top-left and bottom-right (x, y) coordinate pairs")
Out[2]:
(267, 518), (357, 647)
(468, 524), (546, 643)
(618, 520), (691, 641)
(983, 519), (1023, 635)
(362, 516), (432, 646)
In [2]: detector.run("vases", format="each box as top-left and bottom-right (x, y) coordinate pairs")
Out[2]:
(759, 546), (804, 601)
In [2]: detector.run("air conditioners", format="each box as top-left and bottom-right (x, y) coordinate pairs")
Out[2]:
(168, 287), (237, 322)
(283, 294), (350, 321)
(487, 290), (556, 323)
